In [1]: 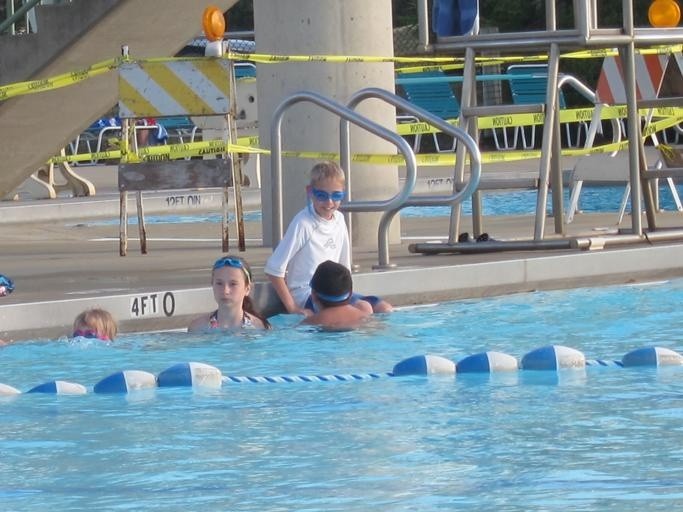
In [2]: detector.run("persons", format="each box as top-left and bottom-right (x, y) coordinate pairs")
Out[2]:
(267, 159), (395, 318)
(70, 305), (122, 346)
(187, 255), (273, 335)
(294, 261), (388, 337)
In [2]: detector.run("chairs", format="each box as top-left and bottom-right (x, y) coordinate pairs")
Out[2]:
(46, 112), (203, 174)
(507, 60), (587, 151)
(395, 66), (505, 154)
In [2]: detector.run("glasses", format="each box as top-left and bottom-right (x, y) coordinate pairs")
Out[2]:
(213, 258), (250, 285)
(311, 186), (344, 201)
(72, 329), (108, 341)
